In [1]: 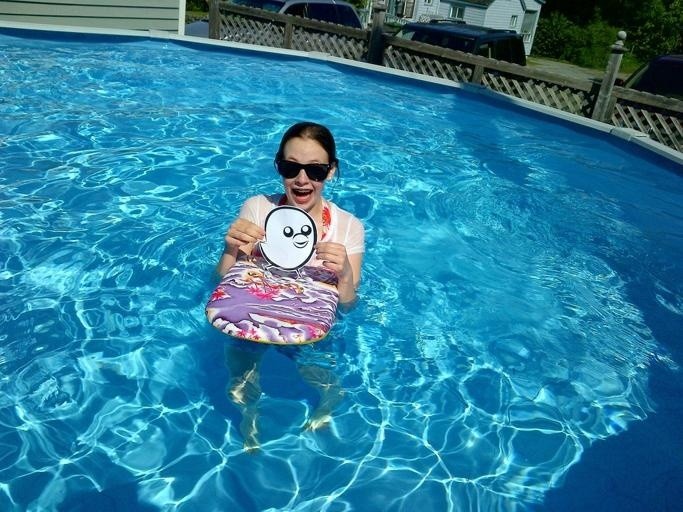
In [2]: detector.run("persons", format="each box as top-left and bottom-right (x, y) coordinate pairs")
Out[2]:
(215, 121), (367, 449)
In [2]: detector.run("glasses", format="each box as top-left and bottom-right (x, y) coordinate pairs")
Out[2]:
(274, 158), (338, 182)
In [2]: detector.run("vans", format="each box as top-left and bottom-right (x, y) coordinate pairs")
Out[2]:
(388, 18), (526, 67)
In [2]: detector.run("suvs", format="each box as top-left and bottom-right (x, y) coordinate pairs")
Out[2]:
(184, 1), (363, 53)
(622, 54), (683, 101)
